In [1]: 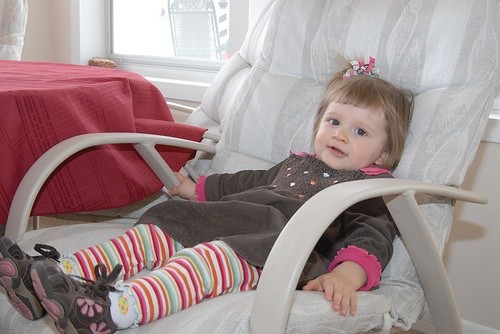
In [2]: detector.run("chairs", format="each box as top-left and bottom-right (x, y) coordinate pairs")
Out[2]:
(0, 1), (500, 334)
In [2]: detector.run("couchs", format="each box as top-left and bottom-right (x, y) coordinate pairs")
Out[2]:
(0, 1), (28, 62)
(34, 0), (280, 228)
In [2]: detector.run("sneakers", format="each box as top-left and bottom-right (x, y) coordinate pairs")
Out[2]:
(0, 236), (60, 320)
(29, 255), (123, 333)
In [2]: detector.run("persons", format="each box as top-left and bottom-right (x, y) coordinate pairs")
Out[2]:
(0, 53), (414, 334)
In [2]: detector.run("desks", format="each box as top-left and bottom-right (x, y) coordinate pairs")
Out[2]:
(0, 61), (209, 234)
